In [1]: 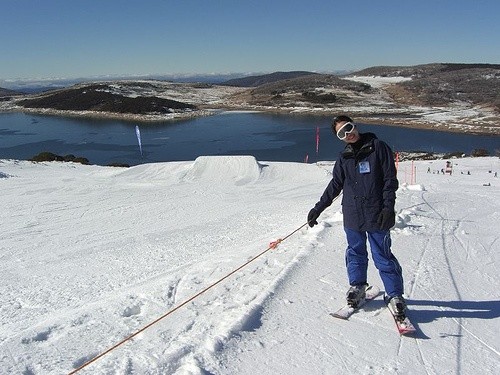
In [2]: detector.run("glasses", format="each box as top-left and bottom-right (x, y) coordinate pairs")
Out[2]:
(336, 122), (355, 140)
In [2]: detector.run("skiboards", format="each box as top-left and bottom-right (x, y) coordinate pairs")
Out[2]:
(330, 286), (416, 335)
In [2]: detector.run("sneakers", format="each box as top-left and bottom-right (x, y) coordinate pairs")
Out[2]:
(346, 284), (366, 308)
(387, 294), (406, 317)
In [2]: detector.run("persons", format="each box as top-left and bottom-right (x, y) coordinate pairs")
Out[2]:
(308, 116), (408, 317)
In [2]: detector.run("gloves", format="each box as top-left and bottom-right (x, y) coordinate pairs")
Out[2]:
(377, 209), (395, 231)
(307, 208), (319, 227)
